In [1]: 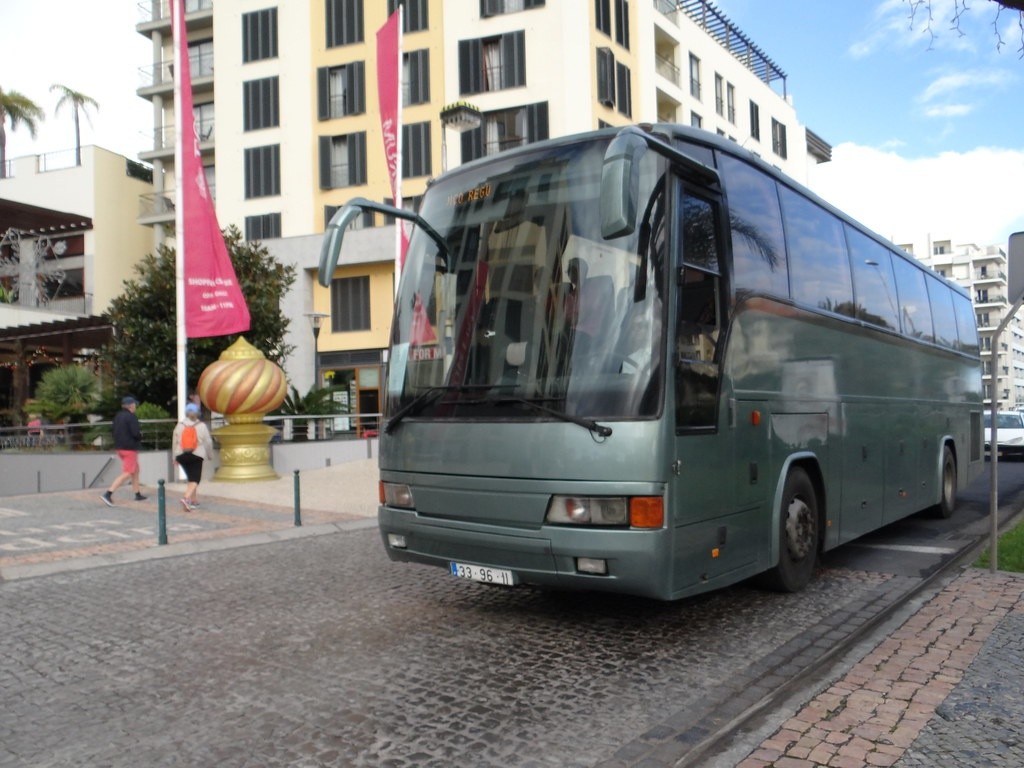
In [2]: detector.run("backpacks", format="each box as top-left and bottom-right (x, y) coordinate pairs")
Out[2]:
(180, 421), (202, 454)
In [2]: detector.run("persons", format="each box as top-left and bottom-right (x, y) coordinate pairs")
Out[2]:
(557, 258), (607, 365)
(100, 397), (147, 507)
(173, 403), (214, 511)
(27, 414), (44, 445)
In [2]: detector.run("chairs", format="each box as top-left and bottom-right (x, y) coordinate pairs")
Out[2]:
(581, 274), (616, 352)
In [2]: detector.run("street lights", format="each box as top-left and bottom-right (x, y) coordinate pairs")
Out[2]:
(303, 311), (331, 439)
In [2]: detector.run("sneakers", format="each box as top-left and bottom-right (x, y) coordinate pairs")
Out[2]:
(190, 500), (200, 509)
(179, 497), (191, 512)
(134, 493), (148, 501)
(98, 492), (116, 507)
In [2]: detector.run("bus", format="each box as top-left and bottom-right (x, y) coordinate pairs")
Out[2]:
(316, 125), (987, 609)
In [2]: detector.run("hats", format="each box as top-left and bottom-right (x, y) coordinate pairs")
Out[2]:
(184, 403), (198, 414)
(120, 397), (141, 405)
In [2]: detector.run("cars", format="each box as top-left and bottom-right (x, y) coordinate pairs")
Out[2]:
(982, 410), (1024, 462)
